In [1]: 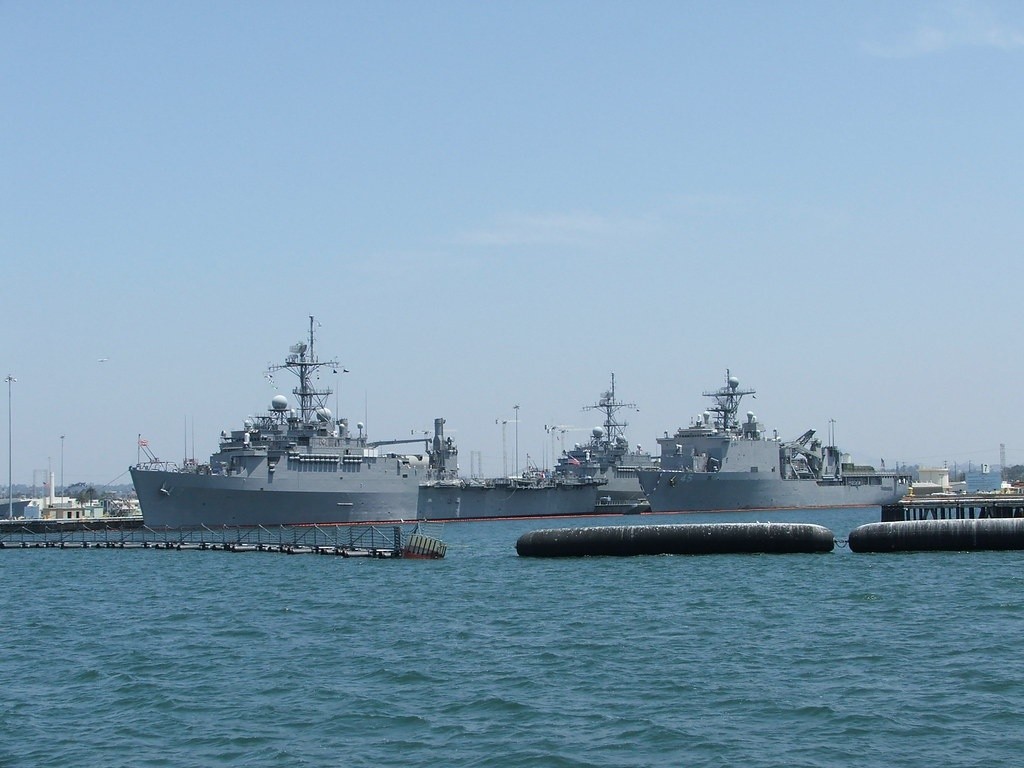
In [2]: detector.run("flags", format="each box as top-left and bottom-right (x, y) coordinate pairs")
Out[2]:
(139, 440), (149, 447)
(568, 454), (580, 465)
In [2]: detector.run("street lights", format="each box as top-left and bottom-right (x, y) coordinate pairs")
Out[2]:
(513, 403), (521, 479)
(829, 418), (836, 446)
(59, 435), (66, 506)
(3, 374), (18, 520)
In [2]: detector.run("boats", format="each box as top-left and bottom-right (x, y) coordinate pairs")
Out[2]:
(128, 313), (1024, 526)
